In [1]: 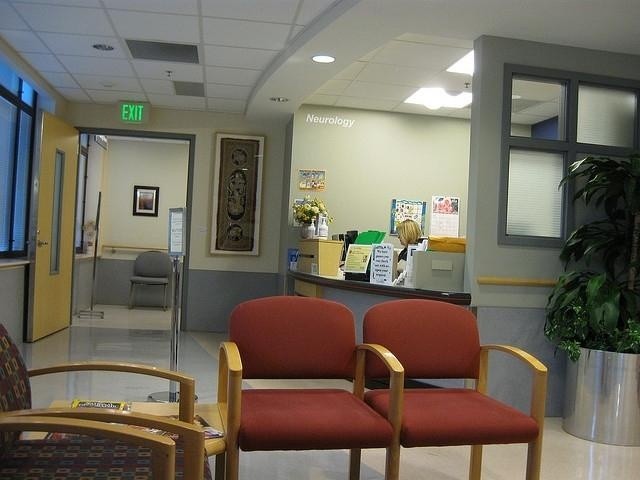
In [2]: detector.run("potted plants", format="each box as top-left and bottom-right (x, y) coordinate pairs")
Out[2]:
(543, 157), (640, 446)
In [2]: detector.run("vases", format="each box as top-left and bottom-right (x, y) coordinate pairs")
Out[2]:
(301, 223), (316, 239)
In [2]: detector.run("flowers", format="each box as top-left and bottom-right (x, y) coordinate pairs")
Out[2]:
(292, 194), (334, 225)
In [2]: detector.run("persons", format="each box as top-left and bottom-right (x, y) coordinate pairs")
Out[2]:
(396, 220), (422, 278)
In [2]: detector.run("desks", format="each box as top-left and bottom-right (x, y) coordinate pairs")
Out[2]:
(29, 398), (227, 480)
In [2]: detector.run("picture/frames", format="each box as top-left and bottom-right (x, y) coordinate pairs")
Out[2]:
(293, 199), (313, 227)
(132, 185), (160, 217)
(210, 133), (265, 256)
(297, 168), (327, 190)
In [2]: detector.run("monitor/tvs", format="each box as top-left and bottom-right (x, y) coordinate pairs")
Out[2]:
(339, 230), (360, 271)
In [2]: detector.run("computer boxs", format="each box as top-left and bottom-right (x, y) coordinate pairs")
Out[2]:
(332, 233), (345, 261)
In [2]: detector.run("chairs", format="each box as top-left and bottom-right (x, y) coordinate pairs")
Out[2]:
(0, 416), (175, 480)
(128, 250), (172, 310)
(217, 295), (405, 480)
(364, 300), (548, 480)
(1, 323), (211, 479)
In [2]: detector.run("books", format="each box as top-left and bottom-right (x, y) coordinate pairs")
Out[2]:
(45, 399), (223, 443)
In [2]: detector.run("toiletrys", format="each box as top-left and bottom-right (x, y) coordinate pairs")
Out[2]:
(307, 219), (316, 239)
(319, 217), (328, 240)
